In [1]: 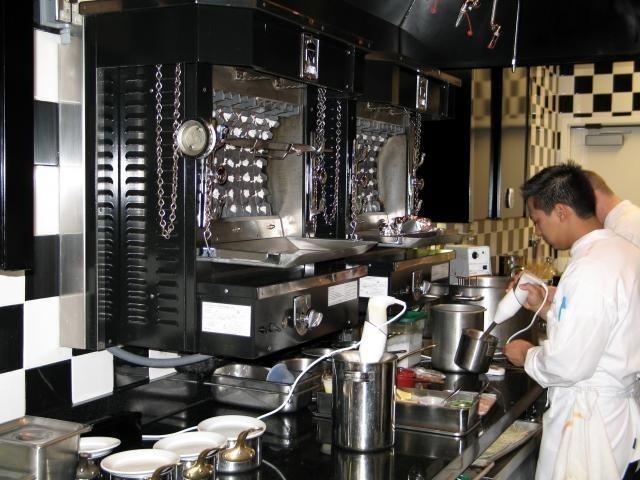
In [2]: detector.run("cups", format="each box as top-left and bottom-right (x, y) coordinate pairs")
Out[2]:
(266, 363), (295, 384)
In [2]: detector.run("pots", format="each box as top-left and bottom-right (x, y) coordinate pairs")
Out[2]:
(456, 275), (539, 361)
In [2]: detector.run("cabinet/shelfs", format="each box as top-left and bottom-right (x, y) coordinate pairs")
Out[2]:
(418, 66), (531, 225)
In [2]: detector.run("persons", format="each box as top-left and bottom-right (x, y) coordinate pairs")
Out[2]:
(501, 161), (639, 480)
(582, 168), (639, 249)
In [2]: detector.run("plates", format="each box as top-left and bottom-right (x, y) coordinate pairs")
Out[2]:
(100, 447), (180, 479)
(77, 435), (121, 461)
(152, 431), (227, 461)
(197, 414), (267, 442)
